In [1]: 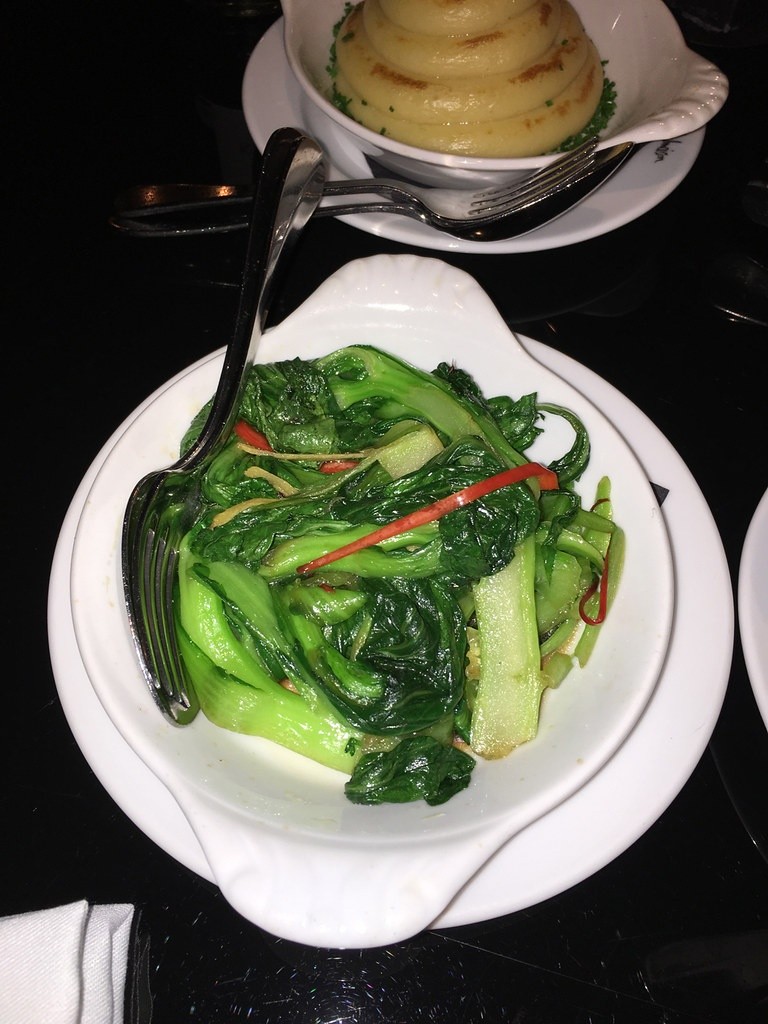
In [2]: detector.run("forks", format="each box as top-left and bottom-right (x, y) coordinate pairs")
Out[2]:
(135, 136), (326, 707)
(112, 135), (598, 223)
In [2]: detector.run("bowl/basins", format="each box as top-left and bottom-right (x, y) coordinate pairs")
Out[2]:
(282, 0), (729, 188)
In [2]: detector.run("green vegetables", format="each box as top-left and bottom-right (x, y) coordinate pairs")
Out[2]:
(146, 344), (627, 807)
(324, 0), (617, 157)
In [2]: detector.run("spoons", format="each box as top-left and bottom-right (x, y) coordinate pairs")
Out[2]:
(126, 143), (636, 241)
(122, 127), (310, 725)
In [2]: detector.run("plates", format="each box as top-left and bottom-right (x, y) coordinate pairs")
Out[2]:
(241, 15), (705, 254)
(47, 318), (734, 927)
(739, 481), (768, 732)
(69, 245), (677, 948)
